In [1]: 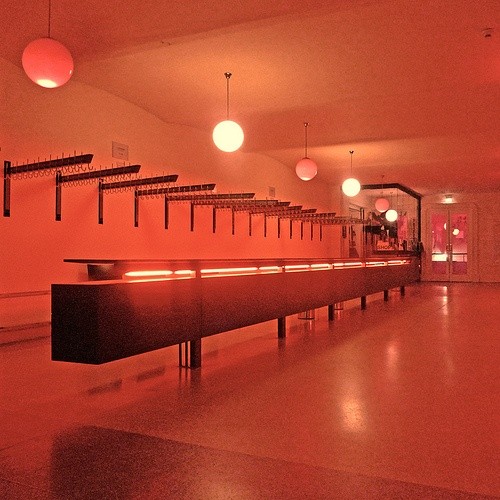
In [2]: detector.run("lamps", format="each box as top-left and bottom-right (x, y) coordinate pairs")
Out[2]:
(445, 194), (453, 203)
(295, 122), (318, 181)
(374, 175), (390, 213)
(212, 73), (245, 153)
(22, 0), (74, 88)
(341, 151), (361, 197)
(385, 192), (398, 222)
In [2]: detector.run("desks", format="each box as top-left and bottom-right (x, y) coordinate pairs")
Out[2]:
(50, 255), (420, 369)
